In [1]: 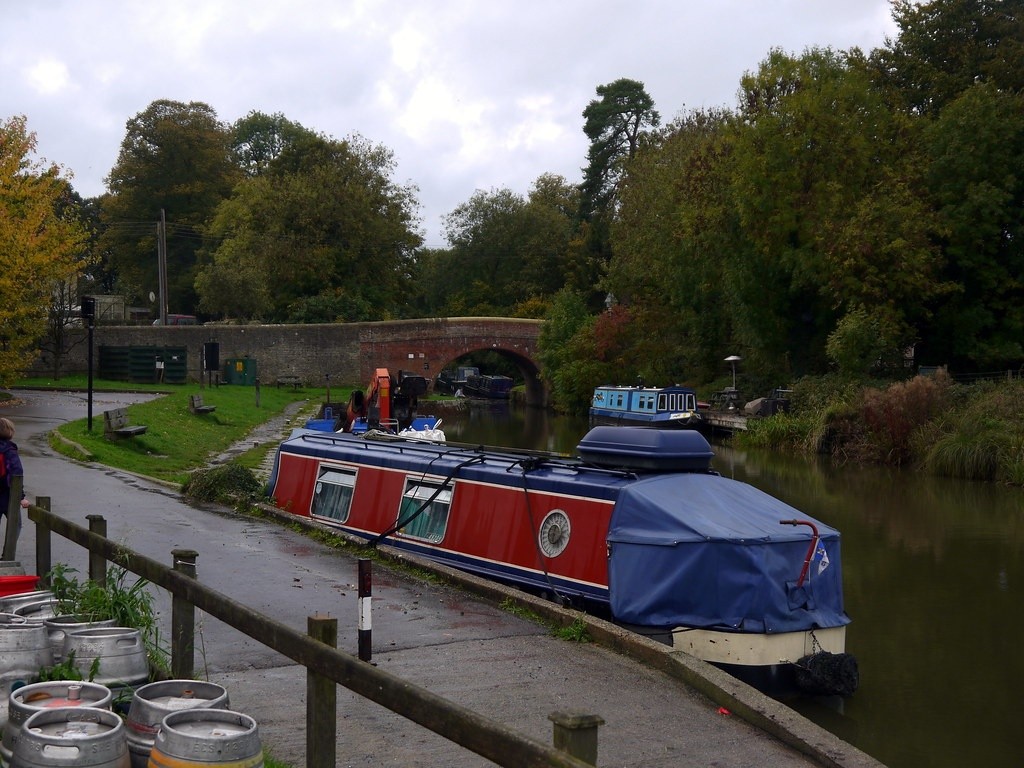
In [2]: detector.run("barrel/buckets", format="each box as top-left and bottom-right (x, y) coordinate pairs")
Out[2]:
(305, 419), (335, 432)
(411, 418), (436, 430)
(0, 575), (269, 768)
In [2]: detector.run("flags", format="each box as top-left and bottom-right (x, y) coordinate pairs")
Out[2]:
(810, 538), (831, 575)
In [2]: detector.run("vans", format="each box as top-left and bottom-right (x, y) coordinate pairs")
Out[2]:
(152, 313), (197, 326)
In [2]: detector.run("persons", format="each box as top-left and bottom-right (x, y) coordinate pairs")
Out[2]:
(0, 417), (32, 539)
(454, 387), (465, 398)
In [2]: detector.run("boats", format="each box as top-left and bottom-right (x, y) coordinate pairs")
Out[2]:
(436, 366), (514, 400)
(589, 386), (698, 427)
(264, 425), (861, 722)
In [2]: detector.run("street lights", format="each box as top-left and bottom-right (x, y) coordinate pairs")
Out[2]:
(724, 355), (744, 387)
(605, 292), (618, 317)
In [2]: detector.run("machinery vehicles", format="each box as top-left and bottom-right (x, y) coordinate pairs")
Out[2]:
(332, 367), (426, 435)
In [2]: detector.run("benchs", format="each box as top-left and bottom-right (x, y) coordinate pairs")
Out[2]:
(104, 407), (148, 441)
(189, 395), (217, 415)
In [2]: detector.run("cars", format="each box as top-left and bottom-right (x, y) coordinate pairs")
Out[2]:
(64, 304), (81, 317)
